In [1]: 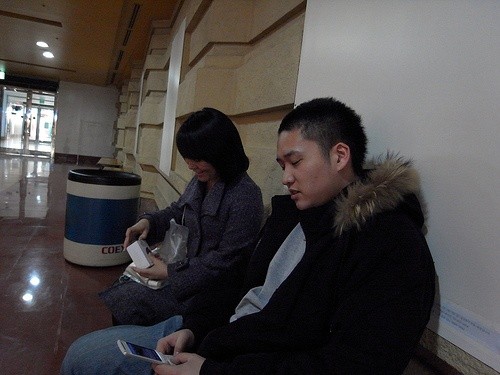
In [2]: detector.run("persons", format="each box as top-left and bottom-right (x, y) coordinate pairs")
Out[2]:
(97, 107), (269, 333)
(57, 96), (438, 374)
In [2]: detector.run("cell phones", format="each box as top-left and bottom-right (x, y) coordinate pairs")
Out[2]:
(117, 339), (181, 365)
